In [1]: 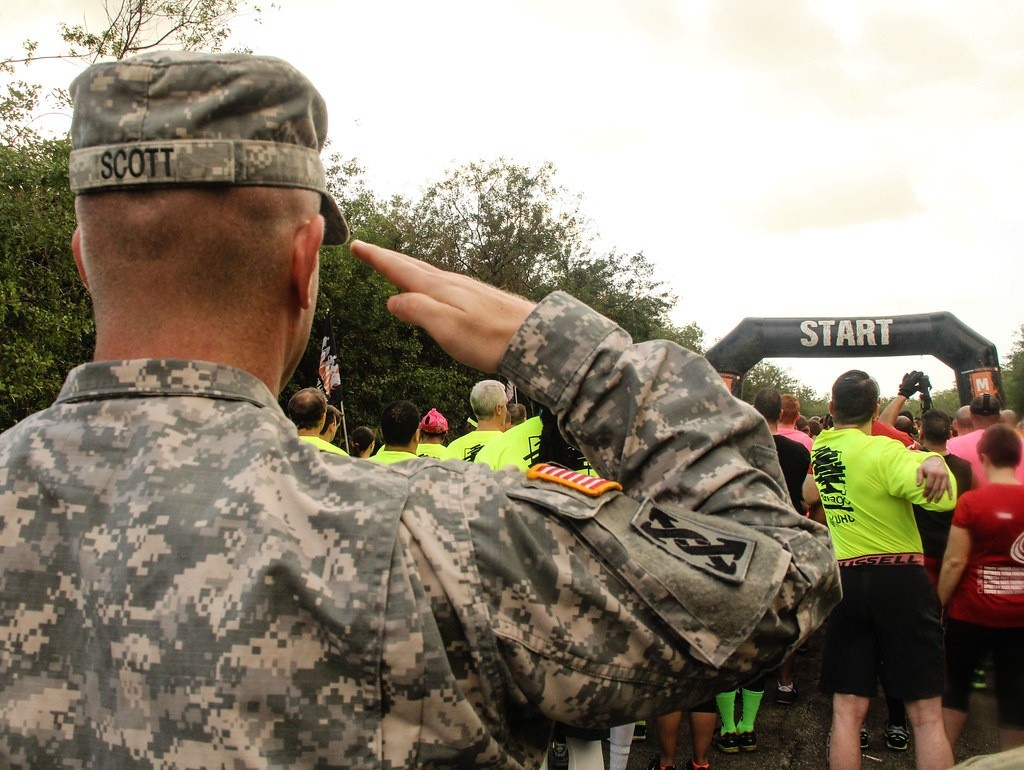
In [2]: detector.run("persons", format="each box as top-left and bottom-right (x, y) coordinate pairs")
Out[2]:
(0, 52), (844, 770)
(284, 368), (1024, 770)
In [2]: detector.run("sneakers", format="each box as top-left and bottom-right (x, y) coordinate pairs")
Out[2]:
(860, 727), (869, 748)
(884, 720), (910, 750)
(776, 680), (798, 704)
(685, 757), (711, 770)
(712, 728), (739, 753)
(647, 755), (676, 770)
(738, 732), (757, 752)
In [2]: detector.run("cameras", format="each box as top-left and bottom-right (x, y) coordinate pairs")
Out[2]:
(917, 375), (932, 413)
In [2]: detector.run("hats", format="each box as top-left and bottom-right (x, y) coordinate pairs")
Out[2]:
(69, 49), (351, 247)
(420, 408), (448, 434)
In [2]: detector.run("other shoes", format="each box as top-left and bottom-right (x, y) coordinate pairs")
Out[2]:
(606, 722), (648, 741)
(551, 739), (569, 769)
(970, 669), (987, 688)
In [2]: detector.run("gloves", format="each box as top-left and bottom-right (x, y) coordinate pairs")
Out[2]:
(899, 370), (930, 398)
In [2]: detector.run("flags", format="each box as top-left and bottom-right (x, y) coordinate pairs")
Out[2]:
(505, 377), (517, 403)
(318, 323), (341, 396)
